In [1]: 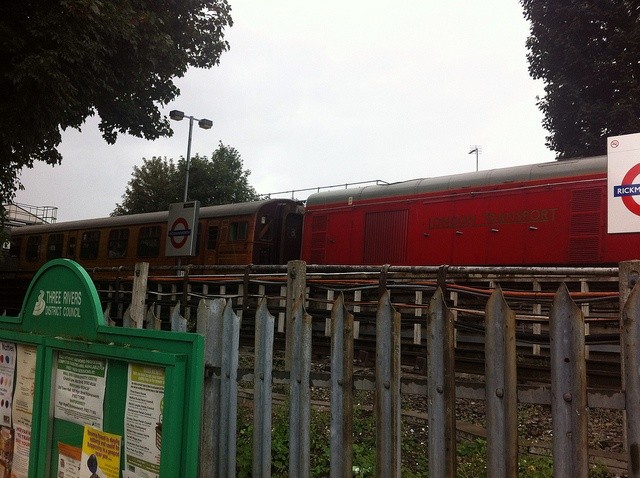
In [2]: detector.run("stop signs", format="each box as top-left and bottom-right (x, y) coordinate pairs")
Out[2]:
(606, 132), (640, 234)
(167, 199), (201, 259)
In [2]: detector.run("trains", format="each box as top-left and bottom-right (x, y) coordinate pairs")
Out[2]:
(1, 150), (639, 378)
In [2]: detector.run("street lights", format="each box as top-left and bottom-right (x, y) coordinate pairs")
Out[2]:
(168, 108), (213, 276)
